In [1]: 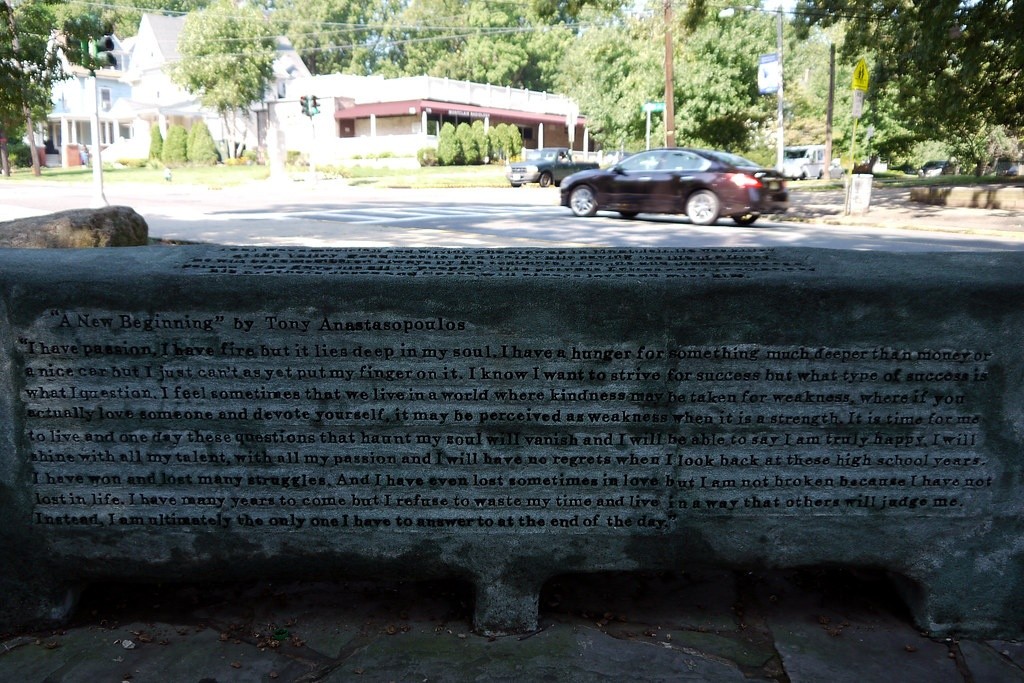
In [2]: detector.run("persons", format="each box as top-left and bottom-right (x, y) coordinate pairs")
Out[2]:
(43, 135), (54, 151)
(80, 143), (90, 167)
(558, 152), (569, 164)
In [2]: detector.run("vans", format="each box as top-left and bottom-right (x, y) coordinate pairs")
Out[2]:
(775, 143), (832, 180)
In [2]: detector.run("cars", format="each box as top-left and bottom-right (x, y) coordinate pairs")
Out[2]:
(560, 146), (790, 226)
(918, 160), (952, 178)
(996, 162), (1020, 176)
(852, 160), (873, 174)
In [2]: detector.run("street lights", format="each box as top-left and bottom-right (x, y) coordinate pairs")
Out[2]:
(717, 5), (785, 176)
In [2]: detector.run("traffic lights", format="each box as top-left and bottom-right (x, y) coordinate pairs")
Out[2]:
(311, 95), (320, 113)
(91, 15), (116, 68)
(300, 95), (309, 113)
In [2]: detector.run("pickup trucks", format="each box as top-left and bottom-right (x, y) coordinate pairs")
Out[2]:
(506, 146), (599, 188)
